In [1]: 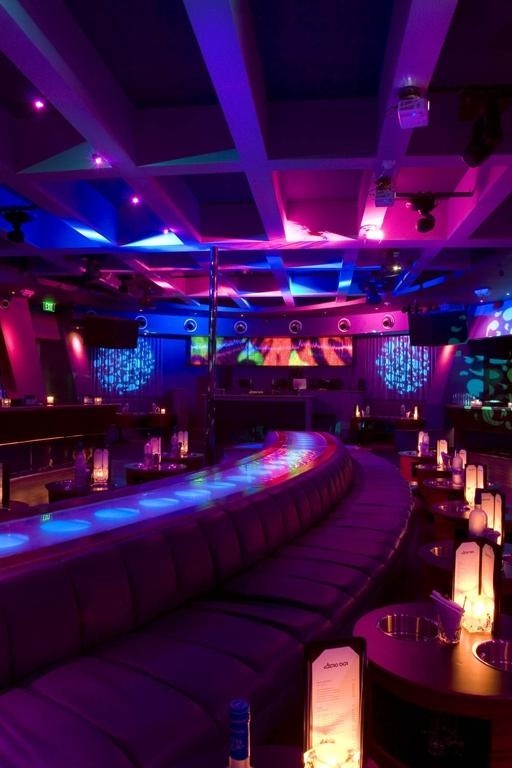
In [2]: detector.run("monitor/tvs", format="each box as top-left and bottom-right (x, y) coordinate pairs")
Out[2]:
(293, 379), (307, 391)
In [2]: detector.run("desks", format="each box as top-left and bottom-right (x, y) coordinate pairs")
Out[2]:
(352, 598), (509, 766)
(419, 536), (511, 583)
(432, 499), (510, 523)
(351, 405), (500, 499)
(200, 392), (315, 449)
(114, 412), (176, 444)
(0, 403), (123, 508)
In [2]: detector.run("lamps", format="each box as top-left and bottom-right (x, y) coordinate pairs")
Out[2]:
(410, 192), (437, 234)
(461, 97), (505, 167)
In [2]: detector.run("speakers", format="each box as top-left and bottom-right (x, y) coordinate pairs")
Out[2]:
(83, 313), (138, 348)
(408, 310), (469, 346)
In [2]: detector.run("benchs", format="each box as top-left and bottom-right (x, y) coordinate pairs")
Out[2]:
(0, 430), (416, 768)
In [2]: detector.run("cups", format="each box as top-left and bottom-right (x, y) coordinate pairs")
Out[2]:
(437, 619), (461, 645)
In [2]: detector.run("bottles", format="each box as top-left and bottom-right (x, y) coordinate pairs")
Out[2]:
(225, 697), (253, 768)
(74, 441), (86, 488)
(452, 443), (463, 484)
(468, 488), (488, 538)
